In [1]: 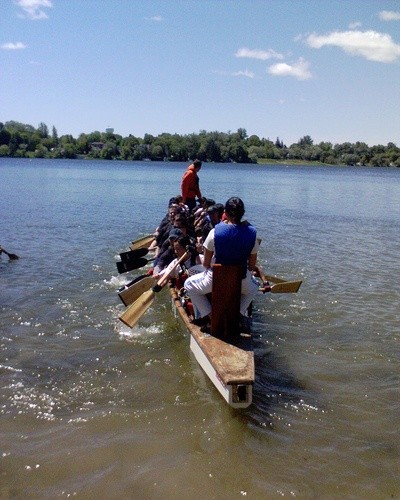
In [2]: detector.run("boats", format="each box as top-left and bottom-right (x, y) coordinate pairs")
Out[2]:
(163, 241), (256, 409)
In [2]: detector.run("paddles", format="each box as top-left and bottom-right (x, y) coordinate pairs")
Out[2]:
(258, 280), (303, 294)
(119, 247), (156, 262)
(128, 234), (156, 250)
(253, 271), (288, 284)
(117, 250), (190, 330)
(115, 258), (155, 275)
(1, 248), (19, 259)
(118, 273), (155, 307)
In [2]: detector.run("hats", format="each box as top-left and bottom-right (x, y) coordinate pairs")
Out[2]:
(169, 228), (183, 238)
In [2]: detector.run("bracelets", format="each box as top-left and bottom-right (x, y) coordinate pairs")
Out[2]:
(263, 281), (269, 286)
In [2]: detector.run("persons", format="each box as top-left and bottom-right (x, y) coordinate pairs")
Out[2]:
(147, 196), (271, 344)
(181, 159), (202, 209)
